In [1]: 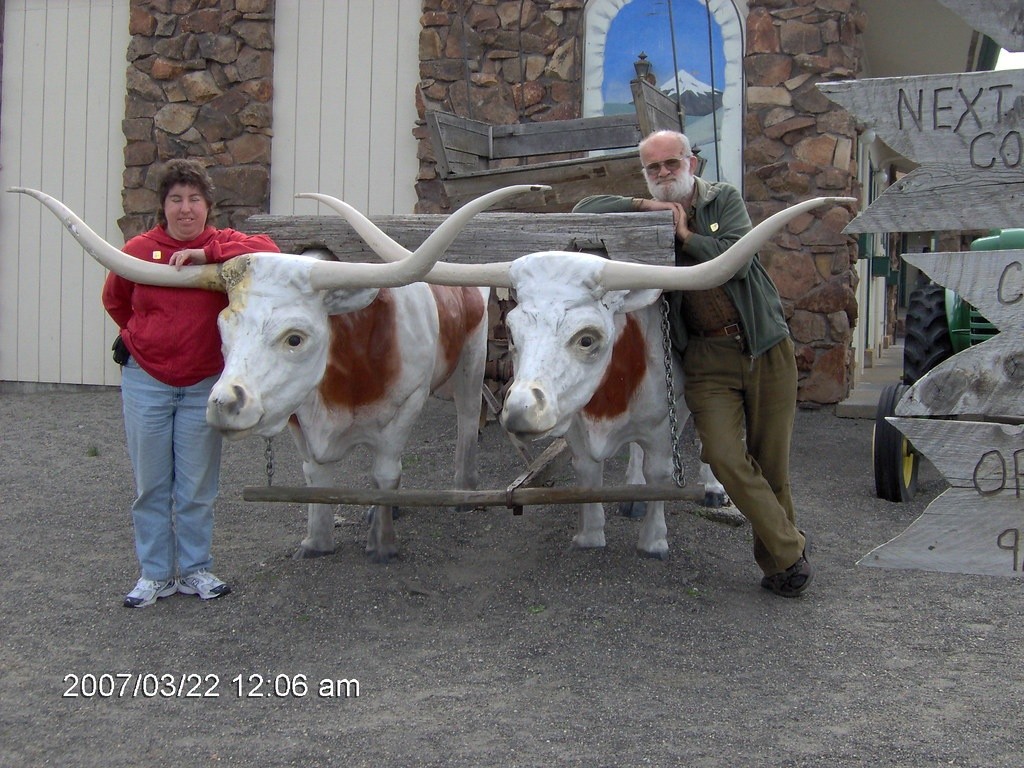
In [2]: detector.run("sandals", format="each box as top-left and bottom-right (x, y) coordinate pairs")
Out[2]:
(760, 574), (803, 597)
(787, 528), (814, 593)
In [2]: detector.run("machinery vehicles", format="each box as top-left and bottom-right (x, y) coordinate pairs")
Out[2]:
(872, 226), (1024, 501)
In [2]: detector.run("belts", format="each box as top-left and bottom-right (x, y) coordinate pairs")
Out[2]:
(702, 321), (742, 337)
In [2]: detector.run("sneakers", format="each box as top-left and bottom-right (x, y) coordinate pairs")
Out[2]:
(124, 577), (178, 606)
(178, 570), (231, 599)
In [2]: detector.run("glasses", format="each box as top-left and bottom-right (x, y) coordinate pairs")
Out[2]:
(645, 156), (690, 175)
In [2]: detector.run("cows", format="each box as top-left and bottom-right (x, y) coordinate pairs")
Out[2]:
(5, 183), (553, 558)
(293, 191), (858, 555)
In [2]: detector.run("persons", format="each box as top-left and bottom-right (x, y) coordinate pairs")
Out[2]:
(100, 158), (283, 608)
(572, 130), (816, 599)
